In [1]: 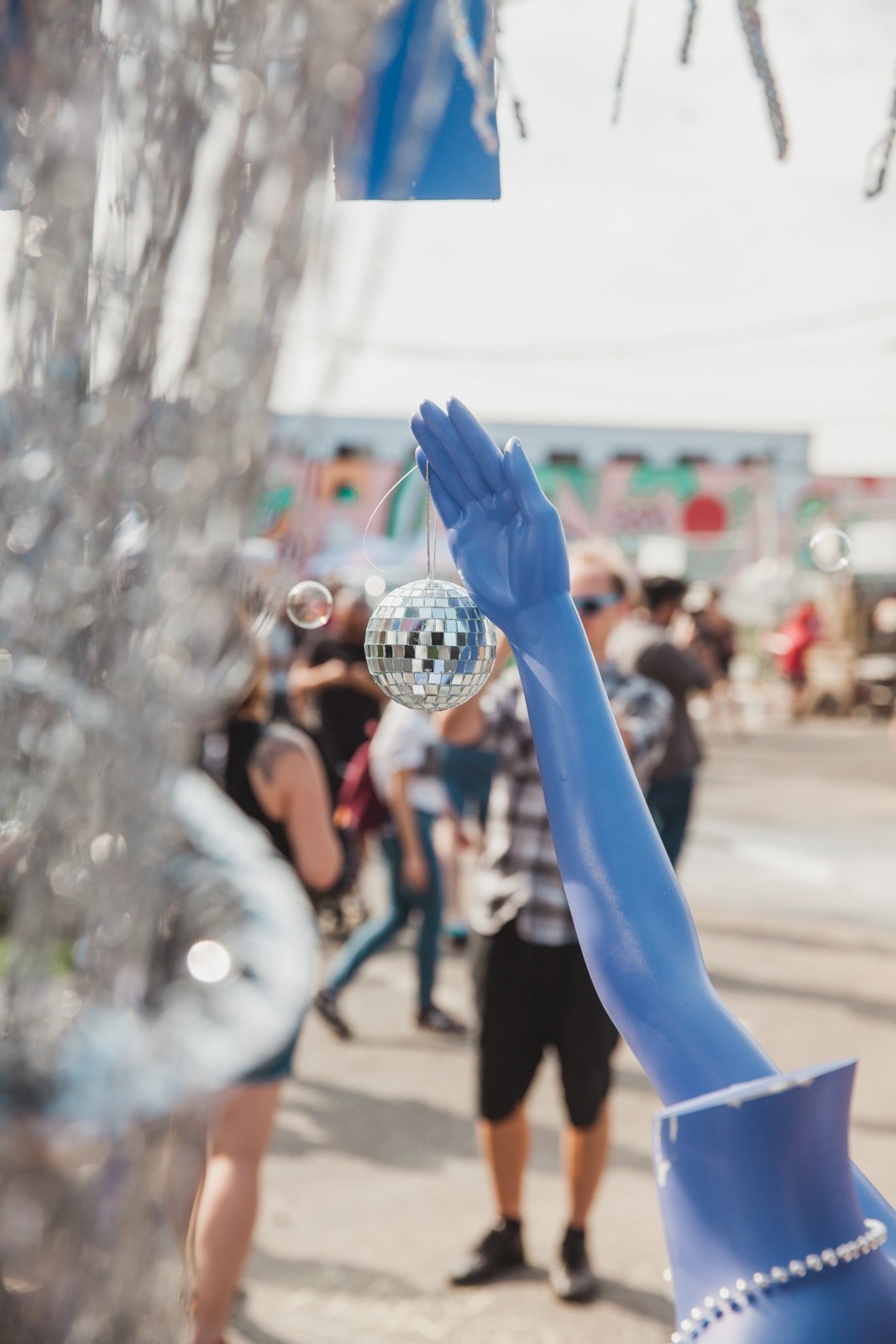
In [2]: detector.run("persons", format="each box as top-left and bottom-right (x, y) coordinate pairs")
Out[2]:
(408, 396), (895, 1343)
(172, 557), (824, 1344)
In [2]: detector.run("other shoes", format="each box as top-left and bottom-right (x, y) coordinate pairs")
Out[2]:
(451, 1223), (525, 1285)
(549, 1242), (592, 1300)
(313, 992), (350, 1040)
(417, 1006), (455, 1030)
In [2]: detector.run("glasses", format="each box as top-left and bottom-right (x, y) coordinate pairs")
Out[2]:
(571, 591), (621, 614)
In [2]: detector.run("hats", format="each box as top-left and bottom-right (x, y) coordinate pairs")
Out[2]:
(643, 575), (685, 609)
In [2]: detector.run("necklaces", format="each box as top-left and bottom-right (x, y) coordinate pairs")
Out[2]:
(668, 1215), (890, 1343)
